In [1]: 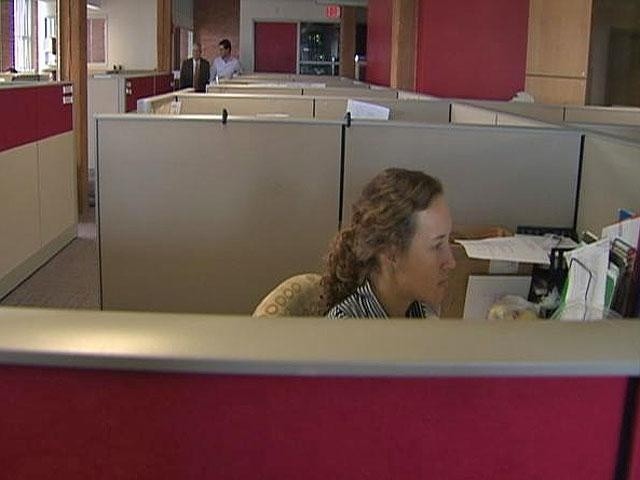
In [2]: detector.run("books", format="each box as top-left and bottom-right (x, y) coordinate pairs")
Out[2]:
(558, 204), (639, 321)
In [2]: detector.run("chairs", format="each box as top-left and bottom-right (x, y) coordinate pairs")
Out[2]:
(252, 272), (326, 317)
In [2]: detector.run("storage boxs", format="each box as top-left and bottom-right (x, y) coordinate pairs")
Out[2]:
(434, 226), (534, 318)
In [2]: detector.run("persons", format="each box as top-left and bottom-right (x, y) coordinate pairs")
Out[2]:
(320, 165), (457, 319)
(209, 39), (242, 84)
(178, 42), (210, 93)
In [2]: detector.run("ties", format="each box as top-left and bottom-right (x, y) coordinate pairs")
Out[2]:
(194, 63), (199, 88)
